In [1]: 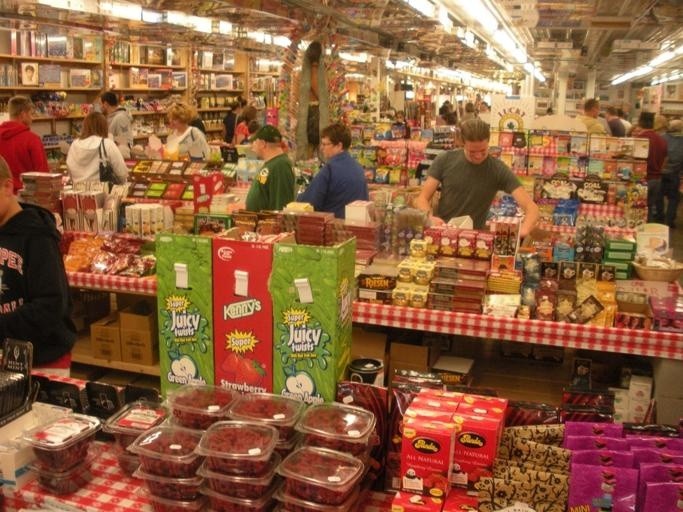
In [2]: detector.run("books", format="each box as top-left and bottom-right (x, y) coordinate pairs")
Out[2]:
(0, 26), (234, 91)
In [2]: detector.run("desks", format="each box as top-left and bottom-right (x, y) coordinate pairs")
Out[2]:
(0, 369), (159, 512)
(63, 265), (683, 436)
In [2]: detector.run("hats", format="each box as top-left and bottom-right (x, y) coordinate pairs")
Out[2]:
(249, 124), (281, 142)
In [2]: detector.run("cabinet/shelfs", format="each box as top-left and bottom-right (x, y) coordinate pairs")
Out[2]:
(0, 0), (507, 186)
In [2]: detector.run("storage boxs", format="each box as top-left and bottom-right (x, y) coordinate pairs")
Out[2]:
(273, 230), (356, 403)
(351, 327), (388, 386)
(90, 314), (122, 363)
(157, 233), (213, 403)
(119, 300), (159, 366)
(388, 342), (438, 386)
(213, 220), (289, 397)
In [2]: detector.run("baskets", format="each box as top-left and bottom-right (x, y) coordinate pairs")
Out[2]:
(632, 257), (683, 282)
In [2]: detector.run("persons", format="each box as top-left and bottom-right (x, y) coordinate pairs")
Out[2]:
(574, 95), (683, 228)
(165, 100), (211, 160)
(244, 124), (295, 212)
(414, 116), (541, 238)
(379, 94), (492, 140)
(0, 91), (45, 196)
(298, 122), (369, 220)
(222, 96), (260, 146)
(0, 153), (79, 378)
(64, 112), (129, 187)
(100, 91), (138, 154)
(188, 113), (205, 136)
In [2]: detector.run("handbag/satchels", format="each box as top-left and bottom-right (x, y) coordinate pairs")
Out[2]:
(100, 161), (113, 182)
